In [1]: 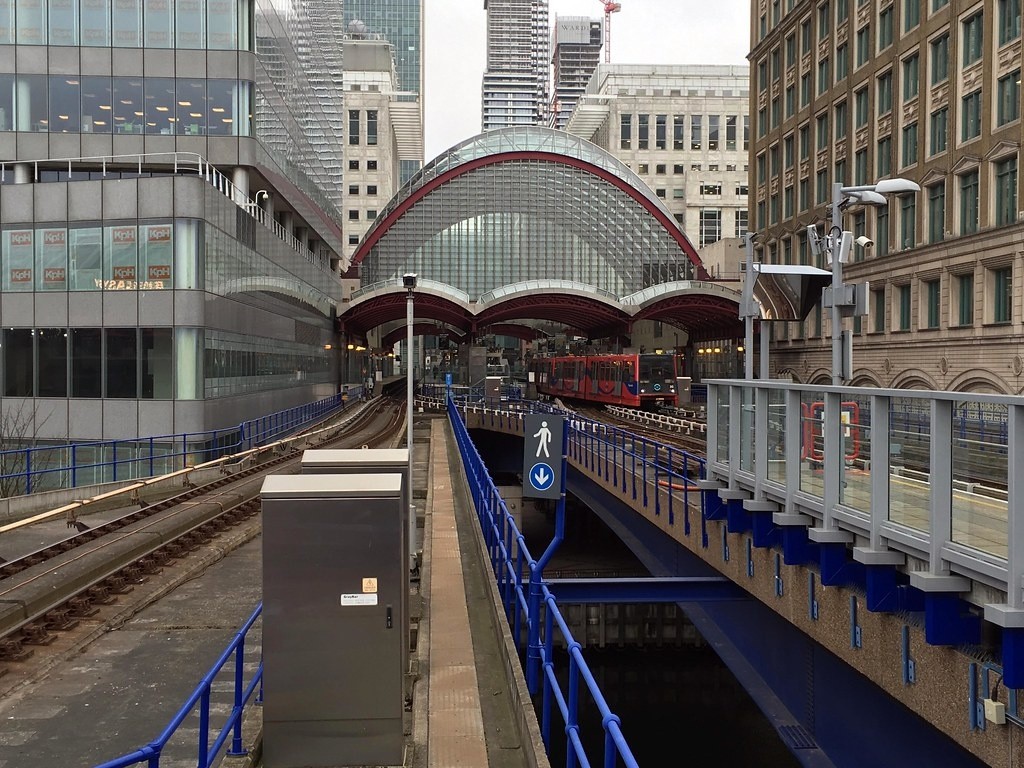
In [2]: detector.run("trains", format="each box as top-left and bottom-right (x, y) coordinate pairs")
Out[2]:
(532, 351), (680, 415)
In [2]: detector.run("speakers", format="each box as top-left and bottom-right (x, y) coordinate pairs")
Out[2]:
(839, 231), (852, 263)
(826, 235), (832, 265)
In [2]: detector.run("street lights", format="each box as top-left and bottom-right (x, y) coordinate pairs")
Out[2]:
(832, 178), (922, 505)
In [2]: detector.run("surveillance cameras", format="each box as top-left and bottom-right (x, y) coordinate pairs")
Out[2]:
(263, 194), (268, 201)
(403, 273), (417, 288)
(857, 236), (874, 249)
(807, 224), (821, 255)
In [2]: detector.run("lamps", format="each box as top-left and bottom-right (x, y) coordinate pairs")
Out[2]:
(723, 344), (735, 354)
(706, 347), (714, 355)
(355, 344), (394, 360)
(698, 347), (705, 356)
(715, 346), (724, 355)
(345, 343), (354, 356)
(737, 341), (745, 352)
